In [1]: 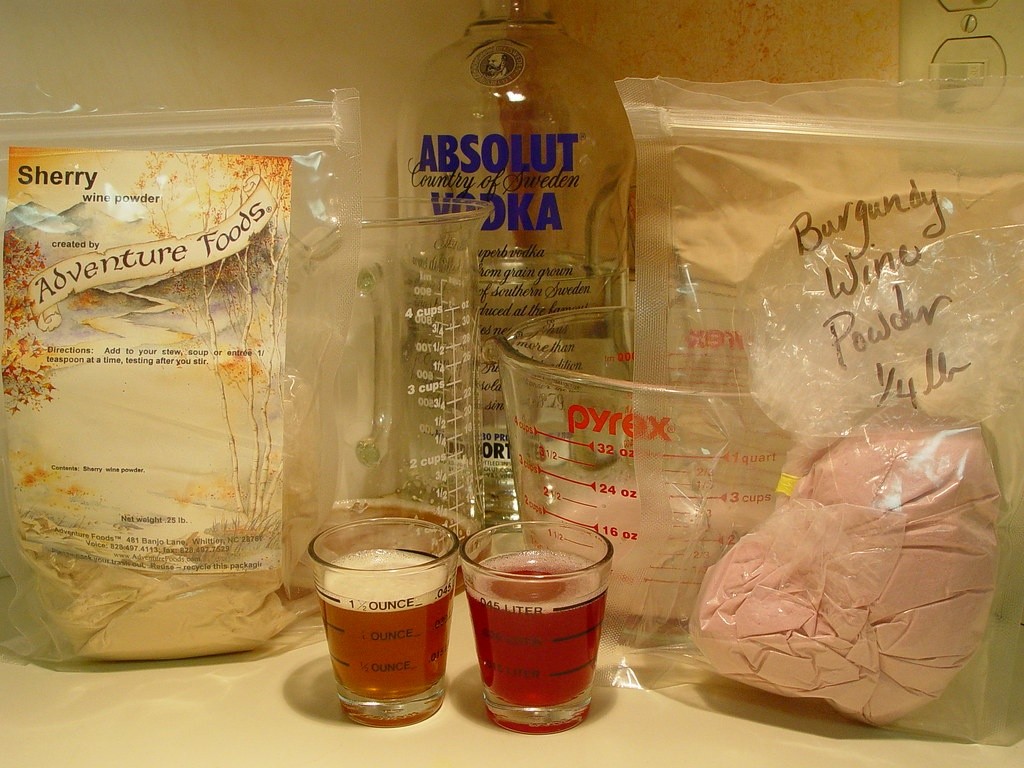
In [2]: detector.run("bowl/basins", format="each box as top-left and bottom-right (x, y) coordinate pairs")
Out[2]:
(482, 305), (796, 617)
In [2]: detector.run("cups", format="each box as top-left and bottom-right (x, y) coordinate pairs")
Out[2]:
(306, 517), (459, 728)
(460, 521), (614, 735)
(358, 196), (496, 595)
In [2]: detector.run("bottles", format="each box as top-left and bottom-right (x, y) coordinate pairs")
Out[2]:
(396, 0), (634, 533)
